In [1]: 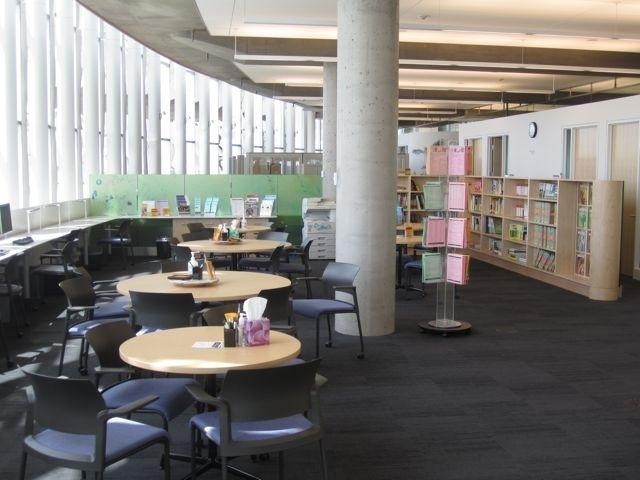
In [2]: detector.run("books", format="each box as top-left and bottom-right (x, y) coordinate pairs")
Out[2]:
(139, 193), (278, 219)
(396, 178), (593, 277)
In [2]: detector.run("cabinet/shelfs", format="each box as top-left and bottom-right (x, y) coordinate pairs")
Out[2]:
(300, 198), (335, 260)
(244, 151), (322, 176)
(397, 173), (624, 303)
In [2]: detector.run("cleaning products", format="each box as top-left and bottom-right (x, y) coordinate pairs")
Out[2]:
(186, 250), (200, 275)
(222, 222), (229, 241)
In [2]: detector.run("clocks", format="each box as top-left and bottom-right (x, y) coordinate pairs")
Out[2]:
(528, 121), (537, 138)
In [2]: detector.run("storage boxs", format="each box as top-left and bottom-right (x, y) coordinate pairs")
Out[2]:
(243, 316), (270, 347)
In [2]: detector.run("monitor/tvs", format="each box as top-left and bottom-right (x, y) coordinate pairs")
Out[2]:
(0, 203), (12, 235)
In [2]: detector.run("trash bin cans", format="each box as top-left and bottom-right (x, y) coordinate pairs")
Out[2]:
(155, 238), (172, 258)
(0, 284), (23, 323)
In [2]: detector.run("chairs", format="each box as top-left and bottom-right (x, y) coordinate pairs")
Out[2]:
(59, 277), (126, 375)
(85, 320), (202, 469)
(17, 363), (172, 480)
(291, 263), (365, 361)
(0, 213), (314, 341)
(189, 358), (326, 480)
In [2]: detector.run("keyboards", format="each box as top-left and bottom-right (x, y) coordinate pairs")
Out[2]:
(13, 236), (33, 244)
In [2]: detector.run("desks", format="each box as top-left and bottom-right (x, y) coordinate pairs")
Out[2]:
(120, 324), (302, 479)
